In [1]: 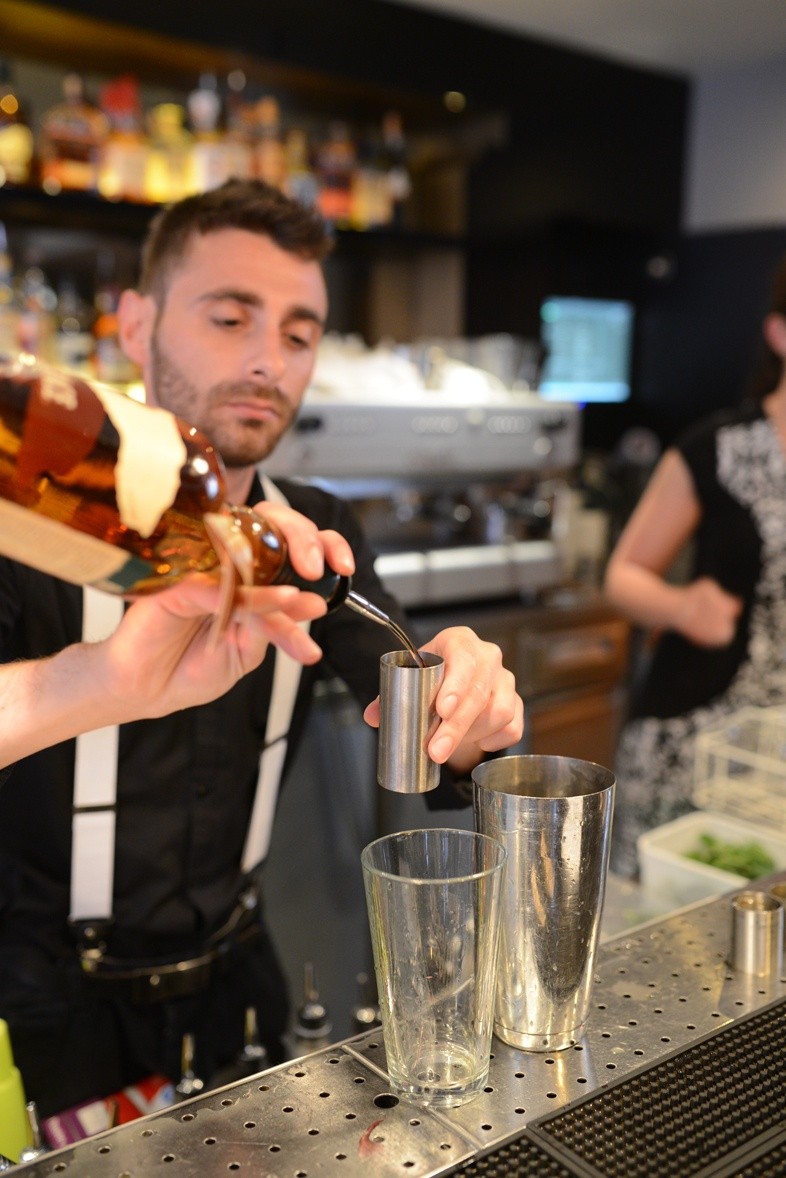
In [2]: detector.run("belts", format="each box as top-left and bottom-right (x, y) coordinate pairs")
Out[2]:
(60, 927), (262, 1001)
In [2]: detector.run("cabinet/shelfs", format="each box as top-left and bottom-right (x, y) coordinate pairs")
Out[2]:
(488, 609), (637, 769)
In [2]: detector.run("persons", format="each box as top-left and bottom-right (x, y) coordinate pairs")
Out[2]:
(601, 275), (786, 888)
(0, 172), (522, 1150)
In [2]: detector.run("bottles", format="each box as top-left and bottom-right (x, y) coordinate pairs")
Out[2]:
(0, 346), (395, 625)
(0, 65), (426, 228)
(1, 237), (144, 383)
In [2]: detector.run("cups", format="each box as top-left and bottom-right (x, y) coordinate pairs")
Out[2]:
(361, 826), (506, 1107)
(377, 650), (446, 792)
(473, 753), (617, 1051)
(728, 884), (785, 976)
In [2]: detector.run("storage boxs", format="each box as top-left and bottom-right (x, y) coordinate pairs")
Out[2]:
(635, 704), (786, 915)
